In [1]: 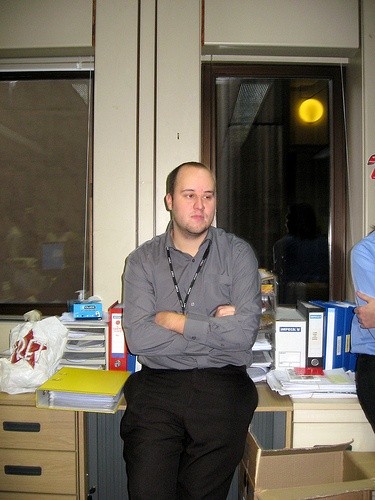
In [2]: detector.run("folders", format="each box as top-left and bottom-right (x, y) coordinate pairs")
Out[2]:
(108, 301), (142, 372)
(35, 367), (132, 414)
(297, 300), (357, 372)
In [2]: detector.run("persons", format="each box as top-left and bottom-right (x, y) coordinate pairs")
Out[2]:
(348, 229), (375, 433)
(118, 160), (260, 500)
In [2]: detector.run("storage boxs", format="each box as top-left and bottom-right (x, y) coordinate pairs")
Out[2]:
(239, 431), (375, 500)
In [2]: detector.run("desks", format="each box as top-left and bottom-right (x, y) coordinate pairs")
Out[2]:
(0, 382), (375, 500)
